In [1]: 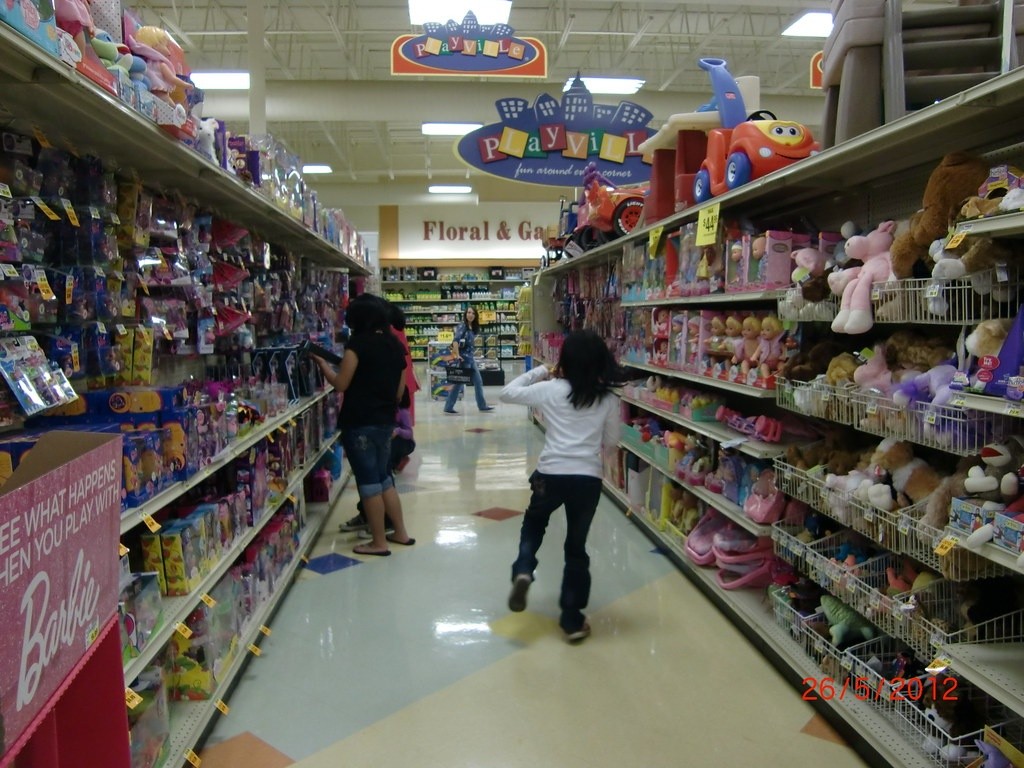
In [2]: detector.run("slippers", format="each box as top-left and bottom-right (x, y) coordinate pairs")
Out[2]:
(354, 544), (391, 555)
(387, 534), (415, 545)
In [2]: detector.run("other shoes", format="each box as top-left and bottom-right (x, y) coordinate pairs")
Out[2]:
(568, 623), (590, 639)
(445, 411), (459, 415)
(480, 406), (495, 411)
(359, 522), (395, 538)
(340, 515), (368, 530)
(509, 574), (530, 612)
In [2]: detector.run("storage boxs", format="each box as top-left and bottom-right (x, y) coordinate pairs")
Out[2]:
(615, 220), (854, 388)
(0, 380), (303, 767)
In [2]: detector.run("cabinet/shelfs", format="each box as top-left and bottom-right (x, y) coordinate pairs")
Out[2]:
(0, 21), (383, 768)
(380, 258), (555, 404)
(530, 67), (1023, 767)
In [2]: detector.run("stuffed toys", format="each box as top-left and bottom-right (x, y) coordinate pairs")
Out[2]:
(773, 146), (1024, 768)
(54, 0), (151, 92)
(189, 116), (220, 167)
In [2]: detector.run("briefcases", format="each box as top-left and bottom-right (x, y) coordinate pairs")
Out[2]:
(446, 361), (475, 384)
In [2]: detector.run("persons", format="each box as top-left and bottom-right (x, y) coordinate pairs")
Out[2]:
(127, 25), (196, 111)
(626, 229), (789, 387)
(335, 304), (421, 540)
(311, 294), (409, 556)
(497, 328), (624, 645)
(442, 306), (496, 416)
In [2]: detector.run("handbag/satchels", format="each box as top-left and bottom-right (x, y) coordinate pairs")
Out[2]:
(449, 333), (465, 355)
(412, 371), (420, 390)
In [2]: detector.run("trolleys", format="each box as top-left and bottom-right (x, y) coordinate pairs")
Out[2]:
(540, 194), (569, 271)
